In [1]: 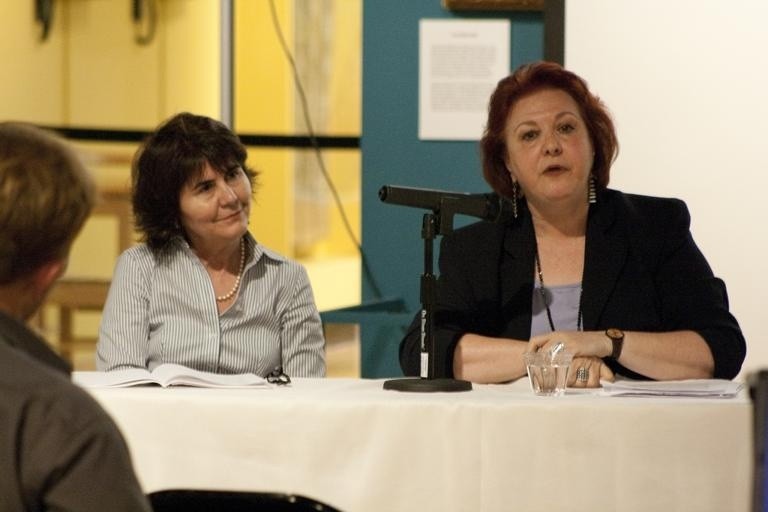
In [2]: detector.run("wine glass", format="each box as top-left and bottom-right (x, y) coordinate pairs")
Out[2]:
(576, 368), (589, 382)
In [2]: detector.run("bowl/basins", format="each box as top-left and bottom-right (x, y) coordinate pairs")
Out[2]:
(536, 248), (582, 331)
(216, 238), (245, 299)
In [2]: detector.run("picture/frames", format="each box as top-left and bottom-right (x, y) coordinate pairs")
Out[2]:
(68, 363), (766, 511)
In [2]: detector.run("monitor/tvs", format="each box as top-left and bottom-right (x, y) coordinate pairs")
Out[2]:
(72, 363), (267, 390)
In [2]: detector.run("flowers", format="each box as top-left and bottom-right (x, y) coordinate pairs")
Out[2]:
(378, 183), (514, 225)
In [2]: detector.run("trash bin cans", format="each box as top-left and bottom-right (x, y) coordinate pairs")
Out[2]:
(605, 328), (625, 361)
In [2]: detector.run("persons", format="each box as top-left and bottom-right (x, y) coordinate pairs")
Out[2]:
(399, 62), (747, 388)
(96, 112), (326, 379)
(0, 120), (154, 512)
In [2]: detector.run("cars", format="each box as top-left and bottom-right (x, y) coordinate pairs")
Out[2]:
(525, 351), (571, 396)
